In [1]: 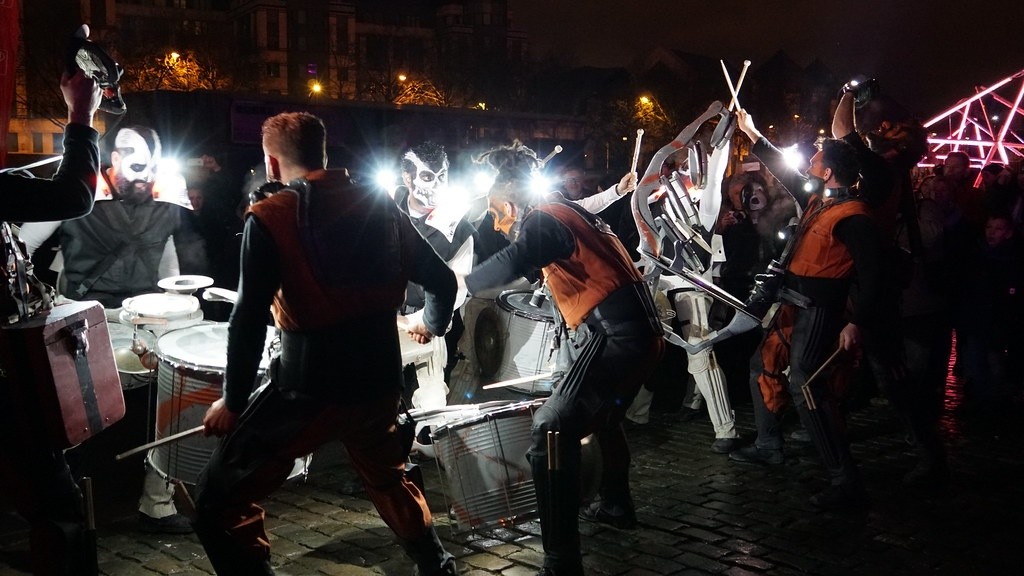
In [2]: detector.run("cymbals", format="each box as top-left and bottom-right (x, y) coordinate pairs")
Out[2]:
(154, 273), (240, 308)
(675, 217), (715, 255)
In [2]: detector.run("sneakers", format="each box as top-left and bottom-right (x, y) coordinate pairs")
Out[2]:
(711, 438), (737, 452)
(810, 481), (868, 507)
(579, 496), (637, 529)
(729, 444), (784, 464)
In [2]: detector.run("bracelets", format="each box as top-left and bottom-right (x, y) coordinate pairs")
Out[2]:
(846, 89), (858, 100)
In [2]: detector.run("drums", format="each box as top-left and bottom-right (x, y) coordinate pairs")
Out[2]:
(635, 111), (764, 324)
(103, 287), (579, 541)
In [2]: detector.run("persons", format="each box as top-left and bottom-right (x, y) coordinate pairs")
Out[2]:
(194, 110), (459, 576)
(0, 68), (1024, 576)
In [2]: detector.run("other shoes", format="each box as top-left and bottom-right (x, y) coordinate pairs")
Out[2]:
(903, 459), (947, 484)
(413, 557), (462, 576)
(139, 513), (194, 534)
